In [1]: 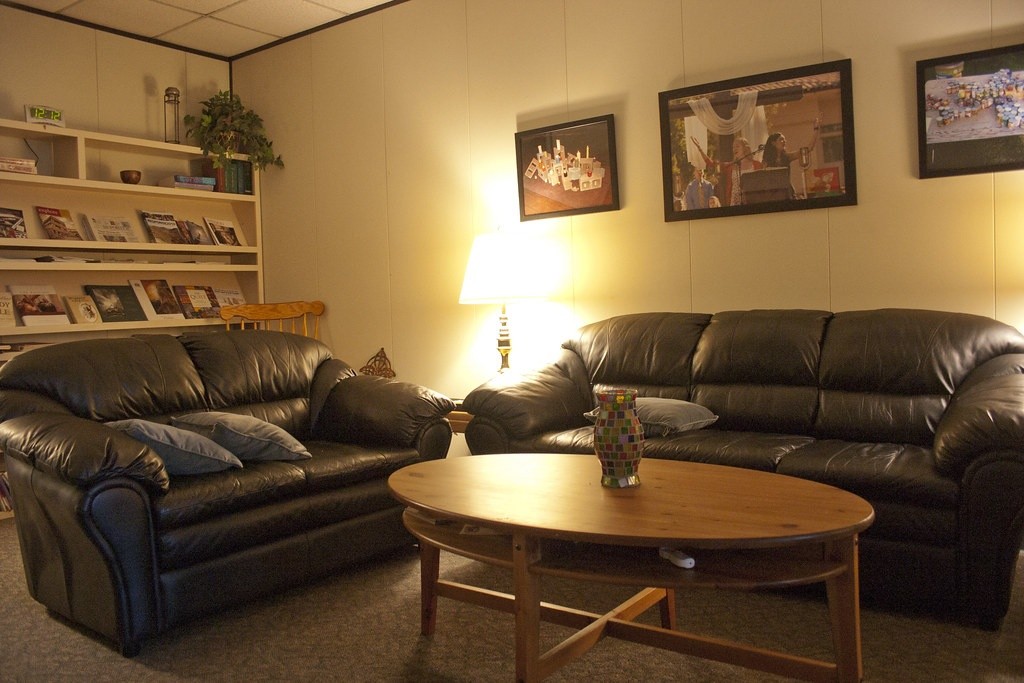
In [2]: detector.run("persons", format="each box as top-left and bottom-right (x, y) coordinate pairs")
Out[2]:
(686, 165), (714, 211)
(691, 135), (761, 207)
(762, 117), (819, 201)
(708, 196), (720, 208)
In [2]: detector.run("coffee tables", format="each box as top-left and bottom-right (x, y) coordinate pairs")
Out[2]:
(387, 453), (874, 683)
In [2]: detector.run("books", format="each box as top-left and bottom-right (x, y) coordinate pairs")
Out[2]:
(0, 156), (248, 360)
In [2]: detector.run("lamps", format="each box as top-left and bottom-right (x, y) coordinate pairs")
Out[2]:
(460, 230), (562, 388)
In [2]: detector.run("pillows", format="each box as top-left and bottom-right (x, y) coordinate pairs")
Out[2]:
(581, 395), (717, 439)
(103, 419), (243, 476)
(170, 411), (314, 461)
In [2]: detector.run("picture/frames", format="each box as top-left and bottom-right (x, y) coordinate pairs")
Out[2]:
(514, 113), (619, 222)
(658, 57), (859, 223)
(915, 43), (1024, 180)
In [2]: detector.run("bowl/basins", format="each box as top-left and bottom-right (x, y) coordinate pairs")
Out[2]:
(120, 170), (141, 185)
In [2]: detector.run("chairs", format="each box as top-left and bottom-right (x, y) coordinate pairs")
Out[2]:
(219, 301), (325, 340)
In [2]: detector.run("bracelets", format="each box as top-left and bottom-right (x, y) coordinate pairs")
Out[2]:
(814, 126), (819, 130)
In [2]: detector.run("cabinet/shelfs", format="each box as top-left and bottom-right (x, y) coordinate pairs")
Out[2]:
(1, 118), (264, 365)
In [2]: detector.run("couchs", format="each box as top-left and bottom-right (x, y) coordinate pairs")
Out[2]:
(1, 331), (457, 660)
(461, 310), (1024, 631)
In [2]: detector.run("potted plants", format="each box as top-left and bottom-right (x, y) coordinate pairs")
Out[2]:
(181, 89), (286, 173)
